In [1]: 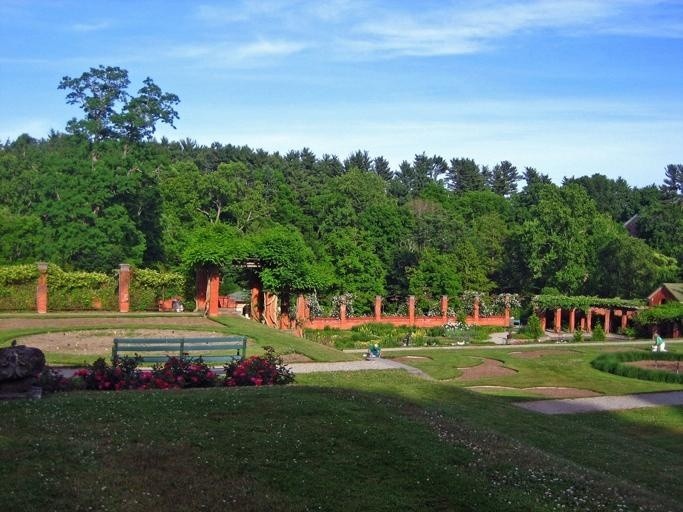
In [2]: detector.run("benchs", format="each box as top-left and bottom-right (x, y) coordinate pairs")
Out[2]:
(110, 335), (247, 375)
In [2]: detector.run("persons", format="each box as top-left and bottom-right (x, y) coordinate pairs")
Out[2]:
(367, 343), (382, 359)
(653, 333), (668, 352)
